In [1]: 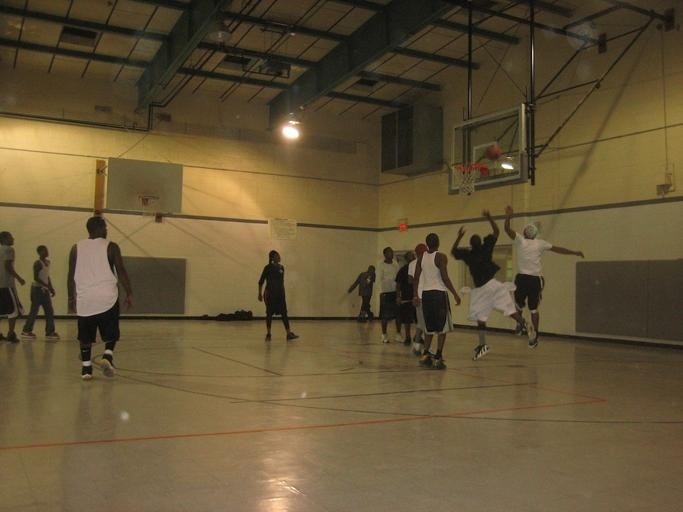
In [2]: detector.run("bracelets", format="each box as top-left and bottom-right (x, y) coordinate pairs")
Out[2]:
(413, 297), (417, 299)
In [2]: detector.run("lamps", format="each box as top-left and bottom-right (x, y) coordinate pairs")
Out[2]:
(500, 154), (514, 171)
(281, 118), (301, 139)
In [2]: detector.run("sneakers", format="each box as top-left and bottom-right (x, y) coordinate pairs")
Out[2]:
(0, 332), (115, 379)
(358, 313), (539, 370)
(265, 334), (270, 341)
(286, 333), (298, 339)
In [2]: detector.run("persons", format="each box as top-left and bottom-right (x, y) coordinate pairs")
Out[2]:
(68, 216), (135, 380)
(451, 209), (537, 361)
(408, 243), (432, 356)
(411, 233), (460, 370)
(395, 251), (417, 346)
(258, 250), (299, 341)
(505, 205), (585, 348)
(0, 231), (25, 343)
(348, 265), (376, 322)
(20, 245), (59, 338)
(376, 247), (404, 343)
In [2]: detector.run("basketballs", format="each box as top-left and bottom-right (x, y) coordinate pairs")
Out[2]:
(486, 143), (502, 160)
